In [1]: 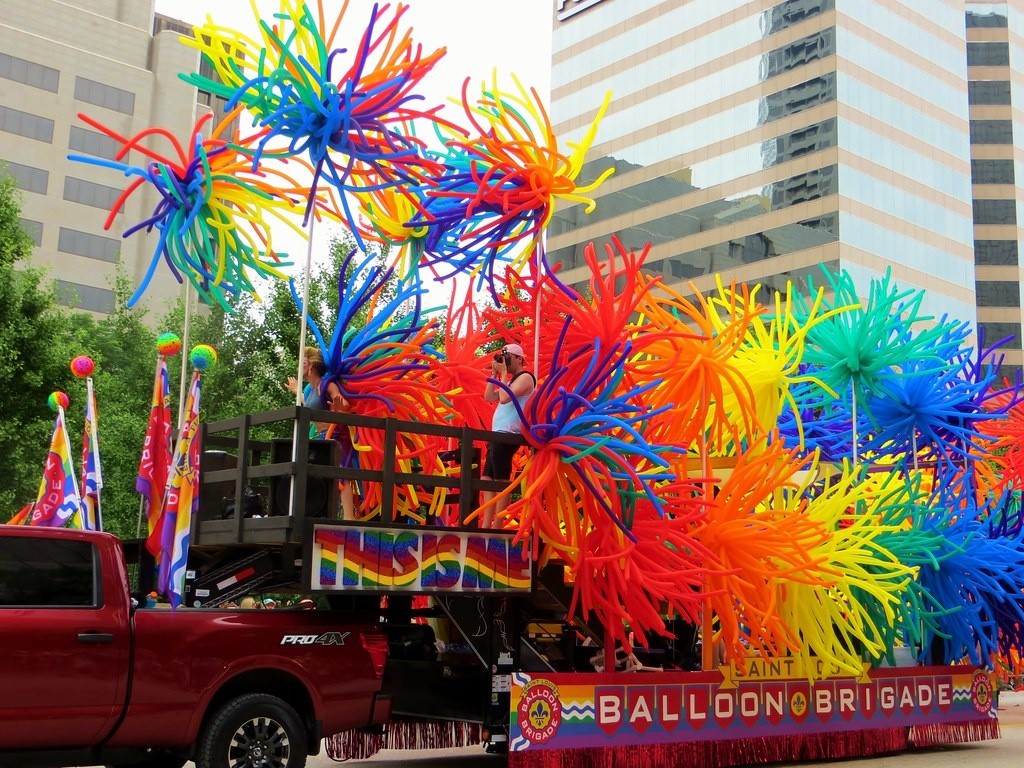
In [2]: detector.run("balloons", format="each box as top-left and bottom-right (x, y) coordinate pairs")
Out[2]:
(62, 0), (1024, 692)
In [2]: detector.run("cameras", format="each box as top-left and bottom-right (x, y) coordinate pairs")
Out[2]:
(493, 349), (512, 366)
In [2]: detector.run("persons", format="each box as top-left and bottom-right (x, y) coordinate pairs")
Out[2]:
(283, 346), (357, 520)
(479, 344), (537, 530)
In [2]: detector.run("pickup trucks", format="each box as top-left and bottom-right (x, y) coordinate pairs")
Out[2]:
(0, 523), (394, 768)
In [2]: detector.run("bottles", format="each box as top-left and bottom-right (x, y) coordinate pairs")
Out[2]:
(145, 591), (158, 609)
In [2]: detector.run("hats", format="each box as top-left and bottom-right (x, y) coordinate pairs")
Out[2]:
(262, 599), (276, 606)
(501, 344), (528, 366)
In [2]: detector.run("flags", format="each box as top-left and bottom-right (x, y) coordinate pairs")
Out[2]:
(6, 354), (203, 606)
